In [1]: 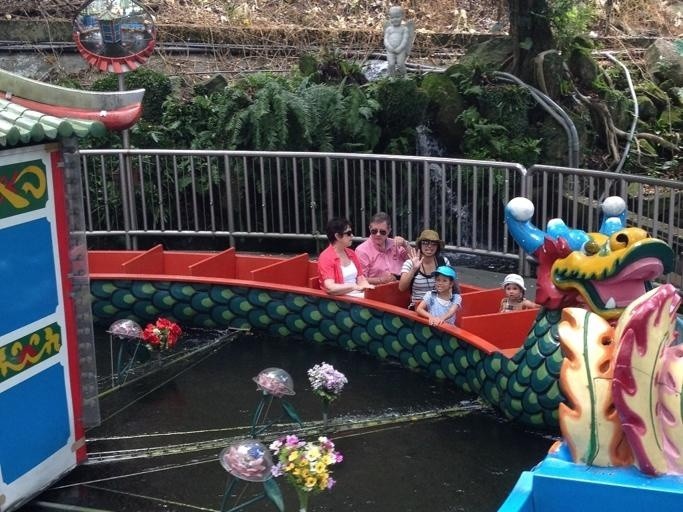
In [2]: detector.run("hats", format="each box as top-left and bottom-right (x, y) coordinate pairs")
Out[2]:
(501, 273), (526, 290)
(431, 266), (457, 280)
(416, 230), (445, 250)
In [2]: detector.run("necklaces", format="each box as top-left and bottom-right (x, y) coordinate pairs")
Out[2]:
(338, 252), (349, 265)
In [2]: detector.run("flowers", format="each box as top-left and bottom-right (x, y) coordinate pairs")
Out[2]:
(266, 431), (345, 512)
(139, 315), (184, 366)
(307, 358), (349, 432)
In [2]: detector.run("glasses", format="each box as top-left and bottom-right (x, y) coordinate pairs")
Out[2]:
(370, 229), (387, 235)
(343, 231), (353, 236)
(422, 241), (438, 245)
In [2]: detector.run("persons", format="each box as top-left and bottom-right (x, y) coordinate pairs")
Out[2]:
(384, 6), (415, 80)
(415, 265), (461, 326)
(317, 218), (375, 298)
(355, 211), (415, 285)
(399, 230), (459, 311)
(498, 274), (541, 312)
